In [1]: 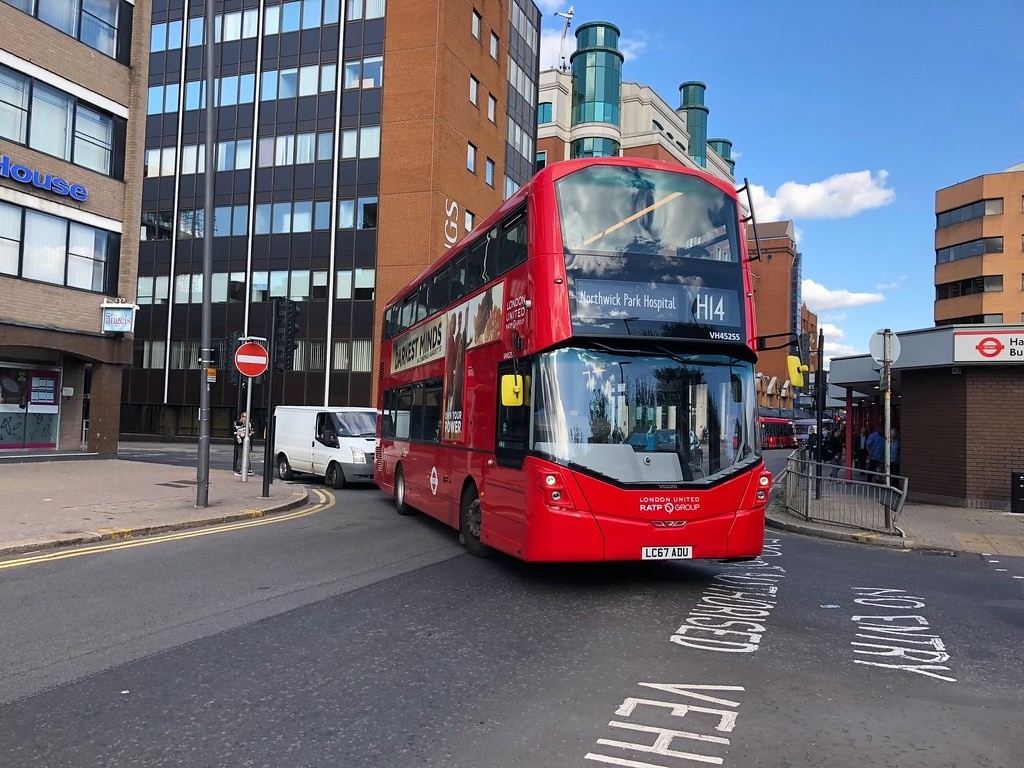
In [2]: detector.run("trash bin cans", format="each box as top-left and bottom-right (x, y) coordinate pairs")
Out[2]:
(1011, 472), (1024, 513)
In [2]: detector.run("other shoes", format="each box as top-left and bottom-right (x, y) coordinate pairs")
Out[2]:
(247, 469), (254, 476)
(234, 471), (242, 476)
(834, 480), (842, 484)
(827, 474), (832, 481)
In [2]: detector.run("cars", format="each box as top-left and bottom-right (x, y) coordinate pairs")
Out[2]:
(621, 432), (654, 452)
(650, 428), (704, 473)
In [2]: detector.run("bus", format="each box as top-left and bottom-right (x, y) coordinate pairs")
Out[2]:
(373, 155), (808, 570)
(727, 417), (840, 451)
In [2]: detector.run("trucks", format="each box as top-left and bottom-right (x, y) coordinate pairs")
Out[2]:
(270, 405), (377, 490)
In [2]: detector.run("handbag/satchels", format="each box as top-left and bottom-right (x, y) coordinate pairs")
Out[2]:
(875, 464), (885, 481)
(821, 447), (835, 461)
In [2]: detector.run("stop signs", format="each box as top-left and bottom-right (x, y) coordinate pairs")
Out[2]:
(234, 342), (269, 378)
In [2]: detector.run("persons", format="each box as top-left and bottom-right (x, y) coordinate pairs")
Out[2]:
(445, 307), (473, 439)
(232, 412), (255, 476)
(535, 395), (579, 416)
(806, 422), (900, 493)
(702, 427), (707, 442)
